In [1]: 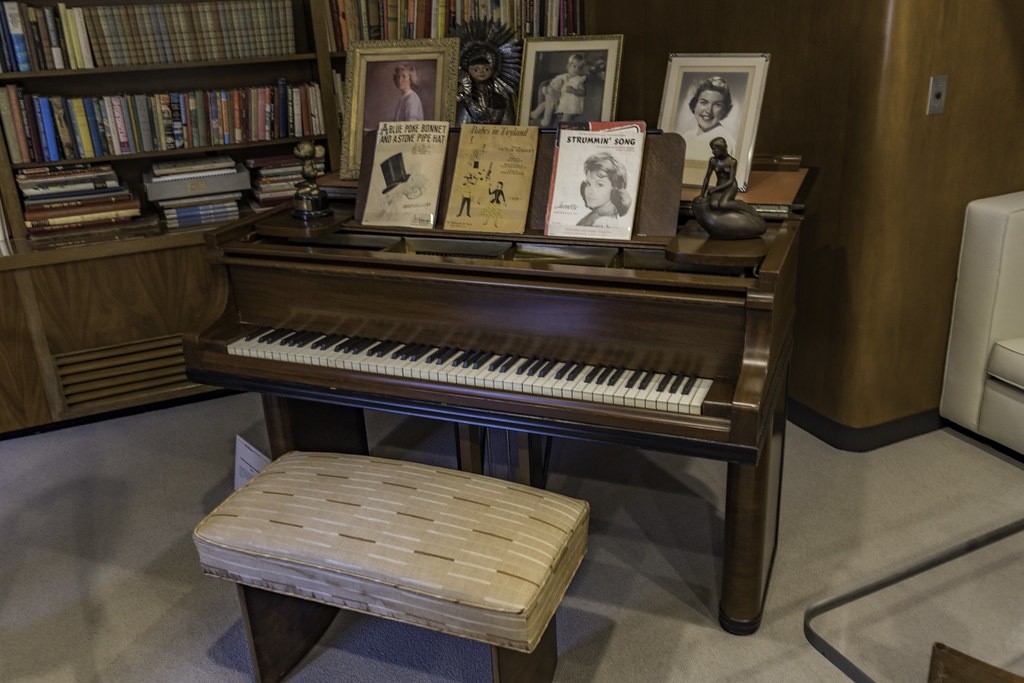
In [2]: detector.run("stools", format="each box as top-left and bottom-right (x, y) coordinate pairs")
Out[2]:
(187, 445), (593, 682)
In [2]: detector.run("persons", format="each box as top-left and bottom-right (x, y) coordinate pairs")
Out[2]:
(673, 73), (741, 161)
(693, 137), (759, 216)
(573, 151), (634, 232)
(454, 52), (510, 127)
(549, 52), (591, 125)
(390, 61), (425, 124)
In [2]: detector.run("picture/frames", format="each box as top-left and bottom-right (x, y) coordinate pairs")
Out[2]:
(335, 36), (461, 187)
(653, 48), (772, 191)
(513, 31), (626, 133)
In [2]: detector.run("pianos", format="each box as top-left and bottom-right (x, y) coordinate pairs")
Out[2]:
(178, 150), (813, 638)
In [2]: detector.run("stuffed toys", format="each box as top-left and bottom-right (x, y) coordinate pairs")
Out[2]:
(531, 76), (564, 127)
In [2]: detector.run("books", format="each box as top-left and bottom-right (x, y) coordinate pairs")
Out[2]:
(360, 120), (450, 231)
(543, 120), (649, 242)
(0, 77), (335, 259)
(443, 122), (540, 236)
(1, 0), (564, 73)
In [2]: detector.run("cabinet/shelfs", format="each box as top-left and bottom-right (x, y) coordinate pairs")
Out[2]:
(0, 0), (347, 439)
